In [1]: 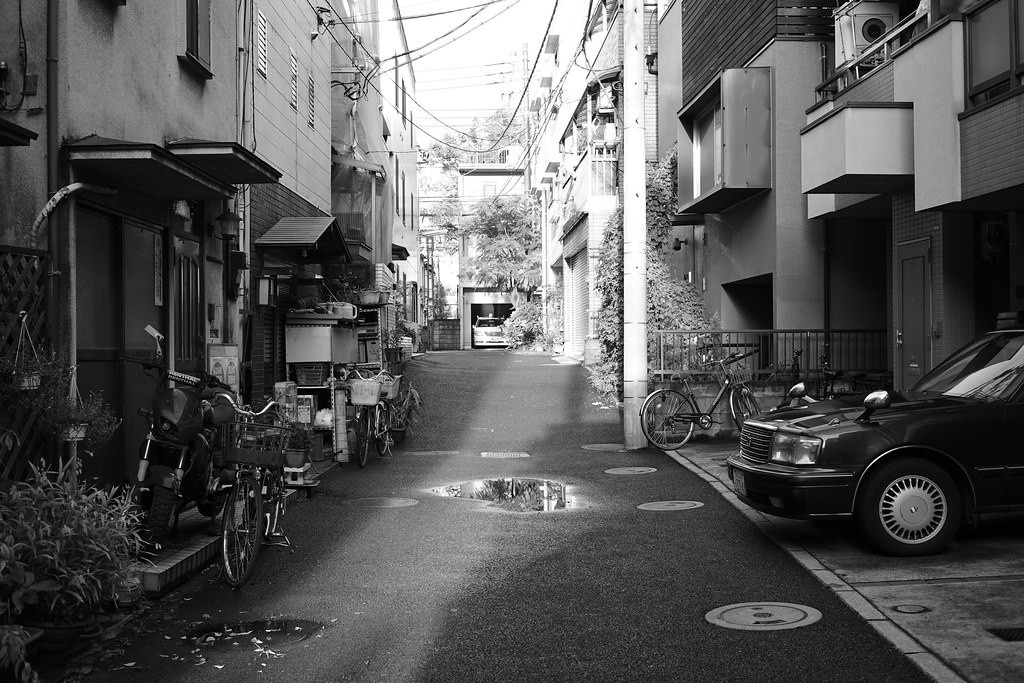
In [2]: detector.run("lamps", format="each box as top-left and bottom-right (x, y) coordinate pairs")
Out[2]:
(207, 208), (242, 241)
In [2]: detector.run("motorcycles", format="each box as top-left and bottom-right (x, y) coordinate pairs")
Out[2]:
(121, 326), (237, 561)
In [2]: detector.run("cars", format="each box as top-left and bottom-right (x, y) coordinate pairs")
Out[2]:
(726, 328), (1023, 557)
(474, 317), (513, 347)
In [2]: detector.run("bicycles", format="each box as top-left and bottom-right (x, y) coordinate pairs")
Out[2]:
(201, 389), (295, 588)
(340, 369), (396, 467)
(638, 350), (762, 450)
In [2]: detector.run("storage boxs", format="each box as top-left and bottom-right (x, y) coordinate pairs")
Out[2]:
(294, 363), (330, 385)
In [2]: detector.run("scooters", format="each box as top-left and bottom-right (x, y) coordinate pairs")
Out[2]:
(767, 348), (872, 411)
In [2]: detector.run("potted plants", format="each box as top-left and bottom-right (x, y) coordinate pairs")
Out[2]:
(384, 326), (405, 362)
(333, 270), (391, 303)
(38, 387), (122, 452)
(283, 423), (314, 468)
(0, 455), (162, 683)
(0, 339), (79, 411)
(389, 382), (423, 443)
